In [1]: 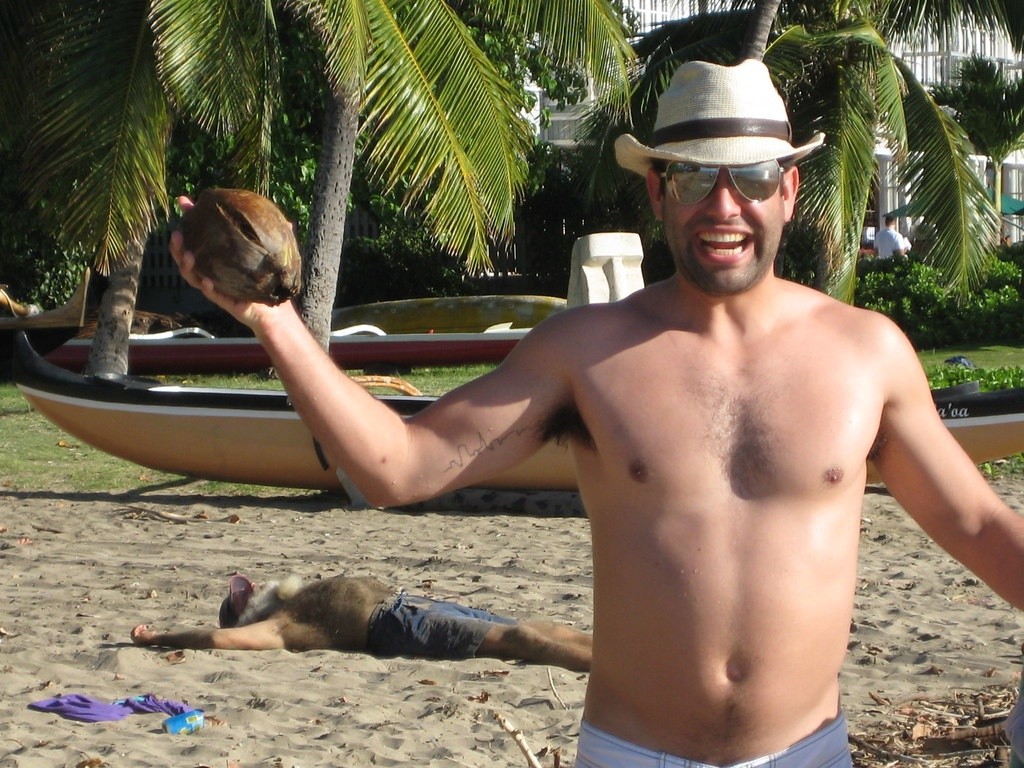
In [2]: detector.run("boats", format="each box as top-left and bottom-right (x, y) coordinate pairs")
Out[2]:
(1, 268), (568, 373)
(9, 330), (1024, 491)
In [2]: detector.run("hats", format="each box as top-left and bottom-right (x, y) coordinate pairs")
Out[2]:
(218, 573), (253, 628)
(613, 59), (825, 175)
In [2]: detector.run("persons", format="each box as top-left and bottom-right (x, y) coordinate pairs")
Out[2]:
(130, 575), (593, 672)
(874, 215), (909, 258)
(169, 59), (1024, 768)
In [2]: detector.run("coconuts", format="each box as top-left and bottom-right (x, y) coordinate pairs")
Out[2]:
(180, 187), (301, 306)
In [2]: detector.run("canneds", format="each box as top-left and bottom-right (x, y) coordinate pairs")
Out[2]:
(162, 710), (205, 736)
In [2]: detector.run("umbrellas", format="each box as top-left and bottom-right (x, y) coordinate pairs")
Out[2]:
(881, 187), (1024, 217)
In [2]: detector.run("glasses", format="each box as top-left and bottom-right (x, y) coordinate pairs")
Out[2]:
(660, 159), (784, 205)
